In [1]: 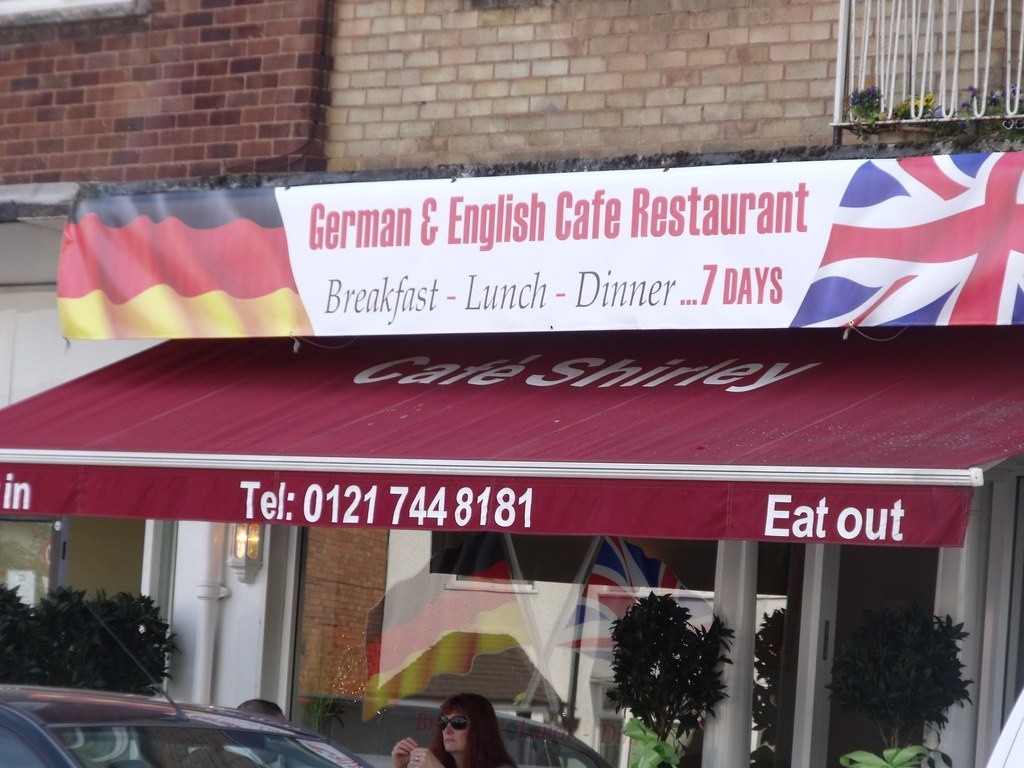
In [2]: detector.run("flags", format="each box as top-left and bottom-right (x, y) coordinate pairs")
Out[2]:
(556, 534), (715, 677)
(362, 535), (525, 722)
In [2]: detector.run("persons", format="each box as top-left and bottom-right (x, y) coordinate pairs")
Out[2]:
(222, 700), (287, 768)
(389, 693), (518, 768)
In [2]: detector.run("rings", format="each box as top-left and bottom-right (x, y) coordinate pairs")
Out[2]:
(415, 756), (419, 760)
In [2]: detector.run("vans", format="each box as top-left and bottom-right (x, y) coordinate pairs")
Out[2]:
(300, 695), (611, 768)
(0, 684), (375, 768)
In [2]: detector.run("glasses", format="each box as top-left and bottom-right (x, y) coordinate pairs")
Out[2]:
(439, 715), (470, 730)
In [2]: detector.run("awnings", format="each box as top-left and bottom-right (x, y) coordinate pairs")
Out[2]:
(0, 326), (1023, 548)
